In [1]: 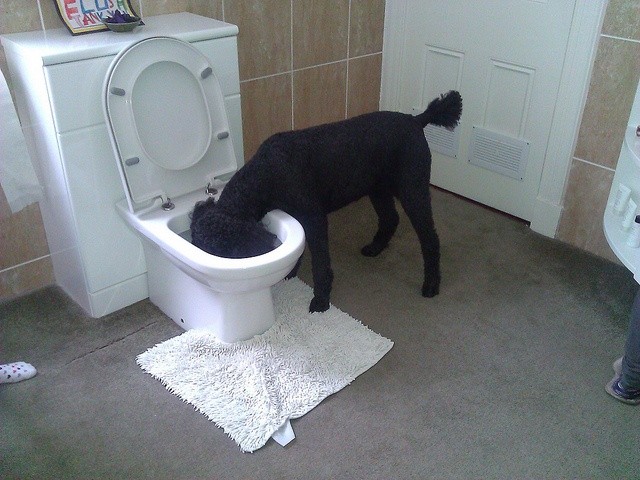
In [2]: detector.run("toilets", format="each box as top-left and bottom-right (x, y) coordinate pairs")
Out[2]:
(102, 36), (307, 342)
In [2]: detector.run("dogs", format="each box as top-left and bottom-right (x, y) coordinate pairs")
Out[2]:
(189, 90), (463, 314)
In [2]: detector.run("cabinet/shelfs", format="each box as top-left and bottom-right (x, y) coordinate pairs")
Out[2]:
(601, 84), (640, 284)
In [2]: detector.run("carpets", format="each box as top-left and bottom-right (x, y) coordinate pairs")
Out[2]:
(134, 276), (395, 456)
